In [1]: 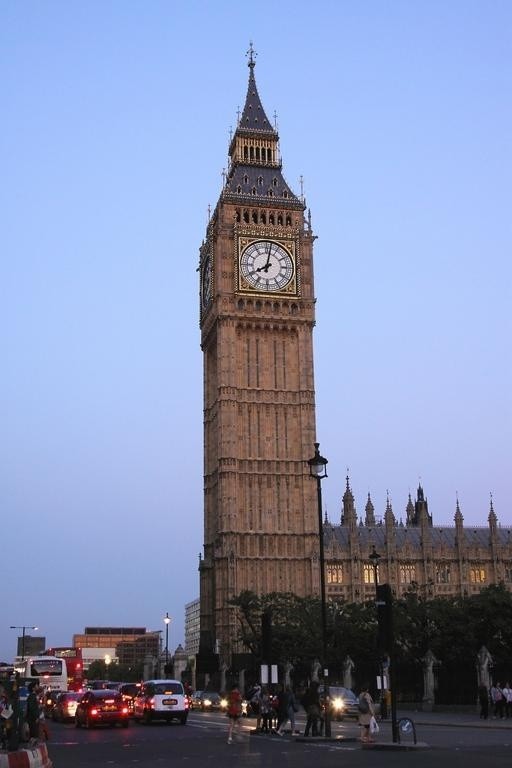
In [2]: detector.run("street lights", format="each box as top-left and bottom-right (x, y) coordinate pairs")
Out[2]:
(163, 611), (173, 679)
(366, 540), (382, 595)
(306, 439), (333, 739)
(9, 624), (39, 666)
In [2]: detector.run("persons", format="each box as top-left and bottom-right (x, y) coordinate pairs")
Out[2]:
(225, 677), (328, 744)
(357, 684), (378, 742)
(0, 667), (52, 751)
(490, 680), (506, 721)
(502, 682), (512, 720)
(475, 679), (491, 720)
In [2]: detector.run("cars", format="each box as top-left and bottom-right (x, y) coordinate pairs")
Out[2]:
(35, 680), (146, 728)
(241, 700), (255, 717)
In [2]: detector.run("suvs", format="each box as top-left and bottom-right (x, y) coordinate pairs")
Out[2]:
(133, 680), (191, 726)
(189, 690), (221, 714)
(316, 683), (361, 723)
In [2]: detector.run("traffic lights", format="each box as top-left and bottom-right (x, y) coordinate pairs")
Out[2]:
(375, 581), (394, 660)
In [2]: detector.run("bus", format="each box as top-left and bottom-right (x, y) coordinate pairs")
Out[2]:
(18, 655), (70, 695)
(40, 647), (83, 691)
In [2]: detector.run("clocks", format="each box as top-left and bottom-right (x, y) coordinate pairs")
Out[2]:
(239, 237), (296, 294)
